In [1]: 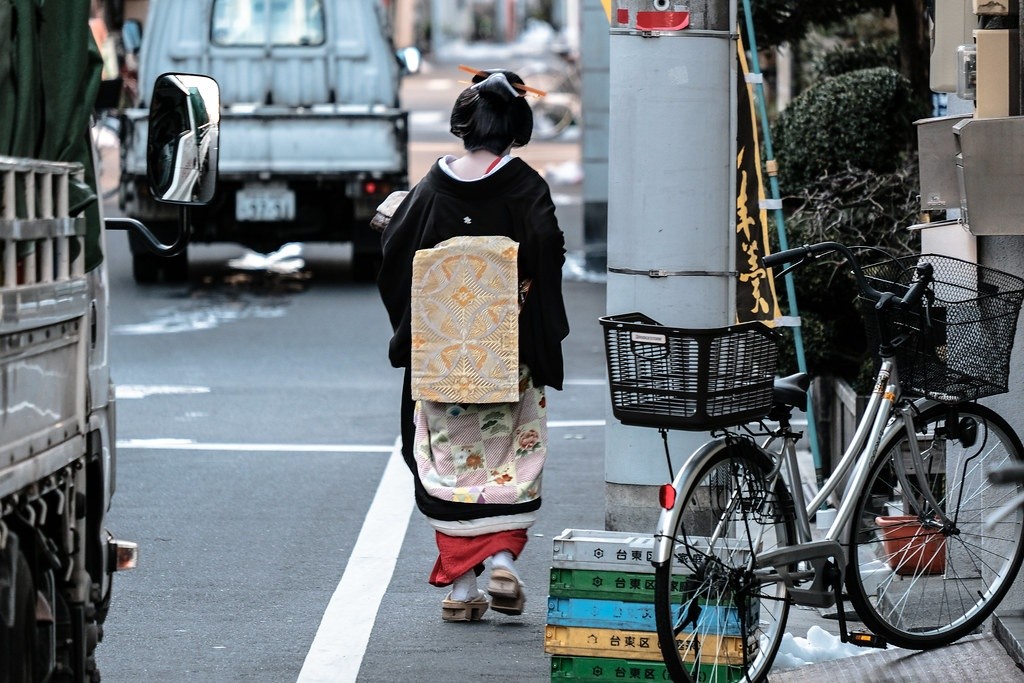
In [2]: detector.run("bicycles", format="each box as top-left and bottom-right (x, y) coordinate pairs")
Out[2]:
(597, 239), (1023, 683)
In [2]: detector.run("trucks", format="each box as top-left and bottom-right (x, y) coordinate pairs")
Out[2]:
(121, 0), (422, 286)
(0, 1), (221, 682)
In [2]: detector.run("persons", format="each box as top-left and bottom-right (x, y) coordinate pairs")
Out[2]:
(371, 70), (571, 622)
(151, 93), (170, 161)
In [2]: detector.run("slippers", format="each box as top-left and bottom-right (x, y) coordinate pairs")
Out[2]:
(487, 569), (527, 618)
(440, 588), (491, 624)
